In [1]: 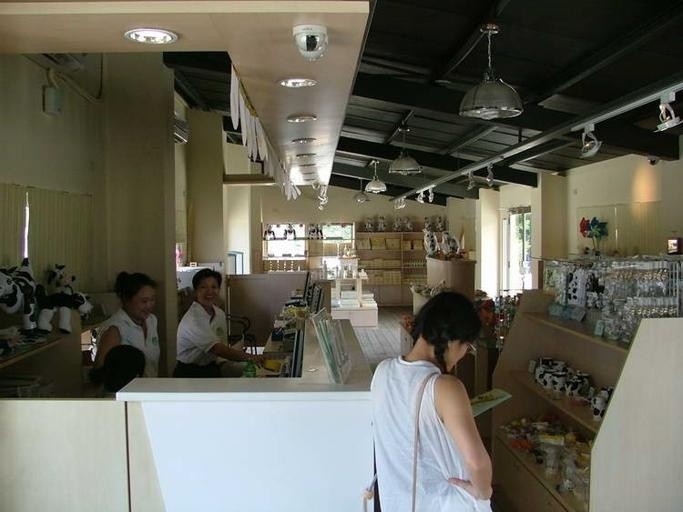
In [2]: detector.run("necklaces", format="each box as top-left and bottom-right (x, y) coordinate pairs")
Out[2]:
(122, 307), (144, 326)
(204, 306), (213, 315)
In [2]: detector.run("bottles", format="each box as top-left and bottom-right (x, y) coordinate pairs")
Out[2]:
(542, 257), (587, 308)
(343, 244), (347, 255)
(242, 359), (256, 378)
(343, 269), (347, 279)
(352, 270), (356, 278)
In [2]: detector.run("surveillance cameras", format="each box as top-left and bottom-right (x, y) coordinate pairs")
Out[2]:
(293, 27), (327, 56)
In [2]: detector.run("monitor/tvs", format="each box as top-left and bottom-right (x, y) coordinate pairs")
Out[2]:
(290, 327), (303, 377)
(310, 283), (323, 314)
(302, 272), (312, 302)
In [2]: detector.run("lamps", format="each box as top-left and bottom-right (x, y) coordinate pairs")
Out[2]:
(353, 181), (370, 204)
(439, 25), (523, 119)
(389, 76), (683, 209)
(365, 161), (387, 195)
(386, 111), (423, 176)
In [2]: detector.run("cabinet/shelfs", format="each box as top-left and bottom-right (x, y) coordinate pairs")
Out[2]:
(490, 290), (683, 511)
(400, 331), (414, 354)
(262, 223), (356, 280)
(356, 232), (427, 306)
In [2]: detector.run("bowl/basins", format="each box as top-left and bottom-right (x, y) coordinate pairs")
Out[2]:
(259, 354), (290, 375)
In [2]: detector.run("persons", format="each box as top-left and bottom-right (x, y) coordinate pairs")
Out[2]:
(173, 269), (282, 378)
(98, 345), (145, 398)
(94, 271), (160, 378)
(370, 292), (493, 512)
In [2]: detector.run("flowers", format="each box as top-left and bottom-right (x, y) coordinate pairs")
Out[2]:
(578, 218), (612, 256)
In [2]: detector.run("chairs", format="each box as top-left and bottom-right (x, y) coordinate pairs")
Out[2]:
(228, 315), (257, 355)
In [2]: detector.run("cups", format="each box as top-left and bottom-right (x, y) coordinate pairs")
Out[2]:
(281, 333), (294, 352)
(527, 355), (608, 424)
